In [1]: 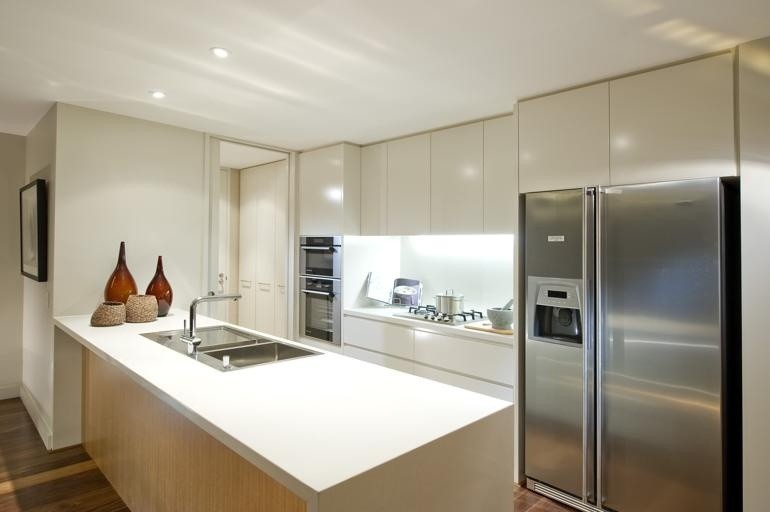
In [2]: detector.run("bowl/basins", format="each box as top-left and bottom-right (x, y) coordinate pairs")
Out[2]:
(486, 307), (513, 329)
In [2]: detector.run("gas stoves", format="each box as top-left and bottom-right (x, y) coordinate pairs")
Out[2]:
(389, 304), (483, 326)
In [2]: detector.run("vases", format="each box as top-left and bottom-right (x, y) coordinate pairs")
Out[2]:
(103, 241), (139, 306)
(144, 254), (173, 318)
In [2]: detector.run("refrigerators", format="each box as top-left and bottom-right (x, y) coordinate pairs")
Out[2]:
(518, 173), (739, 510)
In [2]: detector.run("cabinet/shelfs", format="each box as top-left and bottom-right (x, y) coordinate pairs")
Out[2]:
(218, 166), (240, 326)
(516, 51), (739, 196)
(296, 141), (362, 239)
(340, 311), (517, 403)
(362, 131), (430, 237)
(430, 112), (516, 236)
(236, 158), (289, 340)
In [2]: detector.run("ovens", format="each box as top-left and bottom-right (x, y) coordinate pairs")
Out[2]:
(298, 236), (342, 346)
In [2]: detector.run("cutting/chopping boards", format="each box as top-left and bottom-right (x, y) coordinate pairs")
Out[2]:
(464, 319), (515, 335)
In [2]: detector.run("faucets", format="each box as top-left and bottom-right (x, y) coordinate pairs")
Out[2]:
(180, 293), (242, 355)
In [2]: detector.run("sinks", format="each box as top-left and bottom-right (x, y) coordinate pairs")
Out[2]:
(196, 340), (326, 372)
(139, 325), (268, 349)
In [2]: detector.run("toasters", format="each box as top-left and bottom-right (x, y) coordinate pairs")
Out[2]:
(391, 278), (424, 308)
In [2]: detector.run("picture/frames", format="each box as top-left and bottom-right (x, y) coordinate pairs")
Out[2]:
(17, 179), (48, 282)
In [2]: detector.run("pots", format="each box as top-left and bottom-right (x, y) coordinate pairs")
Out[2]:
(430, 289), (464, 313)
